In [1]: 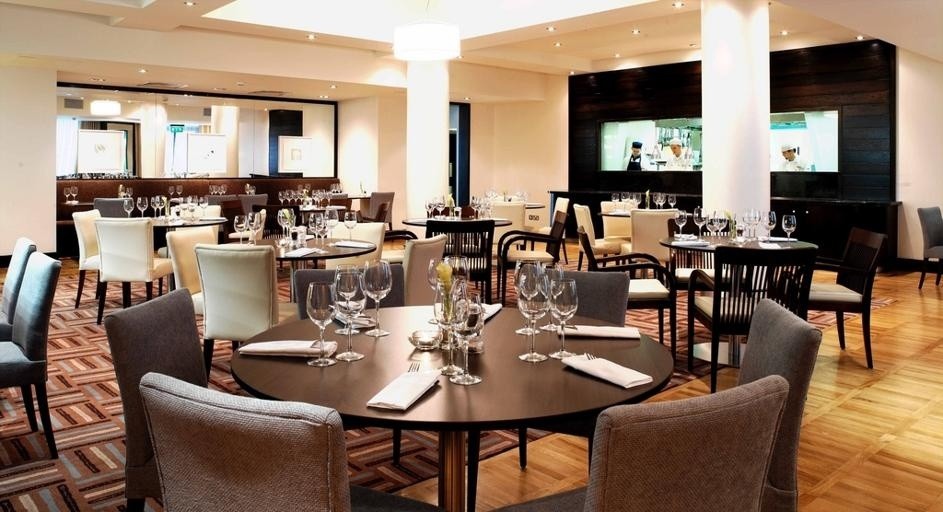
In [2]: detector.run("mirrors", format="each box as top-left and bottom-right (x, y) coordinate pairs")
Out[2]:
(57, 81), (339, 181)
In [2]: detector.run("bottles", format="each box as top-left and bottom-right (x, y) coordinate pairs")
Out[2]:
(503, 192), (506, 202)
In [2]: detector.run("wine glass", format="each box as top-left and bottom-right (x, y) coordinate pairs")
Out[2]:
(62, 185), (78, 205)
(515, 192), (527, 202)
(671, 207), (798, 248)
(208, 183), (256, 196)
(512, 257), (580, 364)
(610, 191), (677, 214)
(232, 206), (356, 252)
(277, 183), (344, 205)
(122, 184), (207, 222)
(425, 193), (493, 221)
(426, 254), (483, 387)
(307, 256), (393, 368)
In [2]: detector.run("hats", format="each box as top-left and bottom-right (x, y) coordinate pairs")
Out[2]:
(633, 142), (642, 148)
(781, 144), (794, 152)
(670, 138), (681, 145)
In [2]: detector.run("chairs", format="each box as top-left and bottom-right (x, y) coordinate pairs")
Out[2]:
(109, 289), (208, 511)
(424, 220), (492, 305)
(917, 205), (943, 290)
(56, 180), (394, 325)
(1, 236), (36, 342)
(600, 201), (644, 266)
(490, 204), (534, 250)
(497, 210), (567, 307)
(628, 209), (676, 278)
(466, 264), (629, 509)
(571, 204), (631, 271)
(294, 263), (406, 465)
(575, 226), (678, 369)
(775, 228), (885, 371)
(524, 197), (569, 264)
(426, 204), (482, 289)
(493, 375), (788, 510)
(688, 247), (818, 395)
(193, 241), (298, 384)
(0, 249), (62, 461)
(733, 298), (827, 511)
(667, 218), (738, 334)
(166, 230), (244, 364)
(401, 232), (449, 305)
(141, 369), (439, 510)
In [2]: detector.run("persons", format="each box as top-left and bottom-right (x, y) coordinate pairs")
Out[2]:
(779, 143), (811, 172)
(620, 140), (650, 172)
(662, 137), (693, 171)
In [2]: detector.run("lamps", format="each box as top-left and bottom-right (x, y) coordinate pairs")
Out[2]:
(393, 0), (461, 62)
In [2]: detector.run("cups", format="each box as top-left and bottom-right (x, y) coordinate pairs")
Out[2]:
(411, 328), (442, 351)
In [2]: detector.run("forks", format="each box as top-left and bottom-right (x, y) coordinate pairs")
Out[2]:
(585, 350), (596, 361)
(406, 362), (423, 373)
